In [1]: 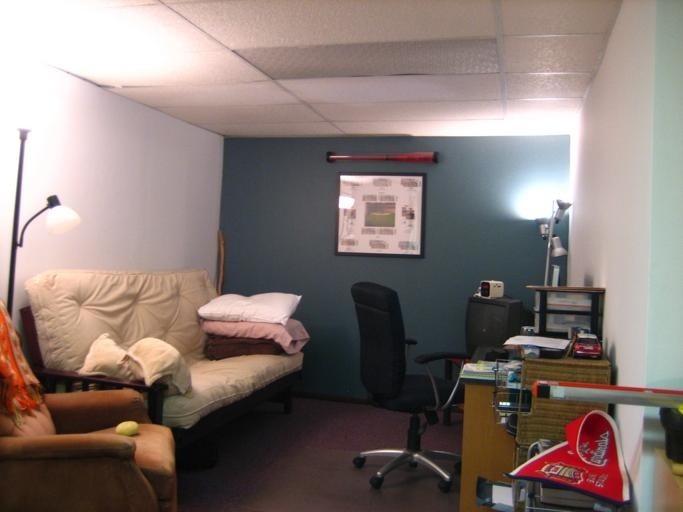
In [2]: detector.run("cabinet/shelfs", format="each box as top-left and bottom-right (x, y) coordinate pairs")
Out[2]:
(525, 285), (605, 338)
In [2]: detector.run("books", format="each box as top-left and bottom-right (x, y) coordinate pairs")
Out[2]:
(461, 360), (508, 382)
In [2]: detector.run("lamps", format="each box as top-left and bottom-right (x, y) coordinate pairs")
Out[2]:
(8, 125), (80, 317)
(530, 193), (572, 287)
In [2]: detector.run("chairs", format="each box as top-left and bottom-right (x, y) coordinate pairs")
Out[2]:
(351, 281), (470, 492)
(0, 298), (178, 512)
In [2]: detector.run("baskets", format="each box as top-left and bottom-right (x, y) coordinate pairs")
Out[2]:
(494, 360), (531, 430)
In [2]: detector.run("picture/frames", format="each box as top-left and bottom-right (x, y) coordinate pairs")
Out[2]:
(334, 171), (428, 258)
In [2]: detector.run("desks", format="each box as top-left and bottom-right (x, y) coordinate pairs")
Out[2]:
(459, 359), (514, 512)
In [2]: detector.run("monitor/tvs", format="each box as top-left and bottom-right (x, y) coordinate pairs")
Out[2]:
(465, 294), (523, 362)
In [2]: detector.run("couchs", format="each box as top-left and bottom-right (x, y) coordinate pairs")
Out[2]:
(19, 305), (308, 425)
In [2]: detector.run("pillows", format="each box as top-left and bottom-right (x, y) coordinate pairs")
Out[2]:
(118, 339), (191, 397)
(200, 320), (310, 354)
(78, 332), (132, 381)
(198, 291), (303, 326)
